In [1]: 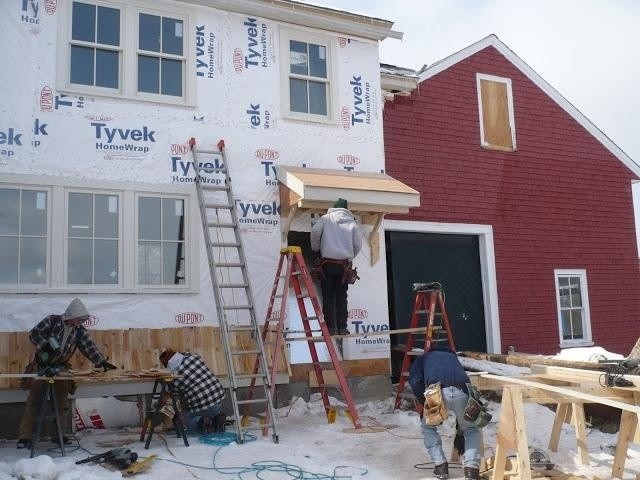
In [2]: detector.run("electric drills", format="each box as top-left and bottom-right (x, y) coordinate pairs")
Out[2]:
(39, 336), (61, 365)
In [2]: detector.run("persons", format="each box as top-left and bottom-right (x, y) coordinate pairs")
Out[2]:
(409, 345), (484, 480)
(310, 196), (363, 336)
(158, 347), (228, 430)
(15, 297), (119, 449)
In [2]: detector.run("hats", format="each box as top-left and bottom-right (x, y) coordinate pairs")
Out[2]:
(333, 198), (347, 208)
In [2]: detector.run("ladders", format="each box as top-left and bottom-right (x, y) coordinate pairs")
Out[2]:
(190, 138), (279, 444)
(395, 289), (458, 414)
(242, 246), (362, 436)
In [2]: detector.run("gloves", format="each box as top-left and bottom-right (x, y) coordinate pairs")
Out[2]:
(41, 343), (56, 354)
(454, 434), (465, 456)
(103, 361), (116, 371)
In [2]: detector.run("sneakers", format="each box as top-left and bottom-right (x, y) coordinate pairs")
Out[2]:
(322, 327), (335, 335)
(17, 439), (31, 449)
(338, 328), (350, 335)
(52, 437), (72, 444)
(464, 467), (479, 480)
(197, 415), (211, 434)
(434, 462), (448, 476)
(212, 413), (226, 433)
